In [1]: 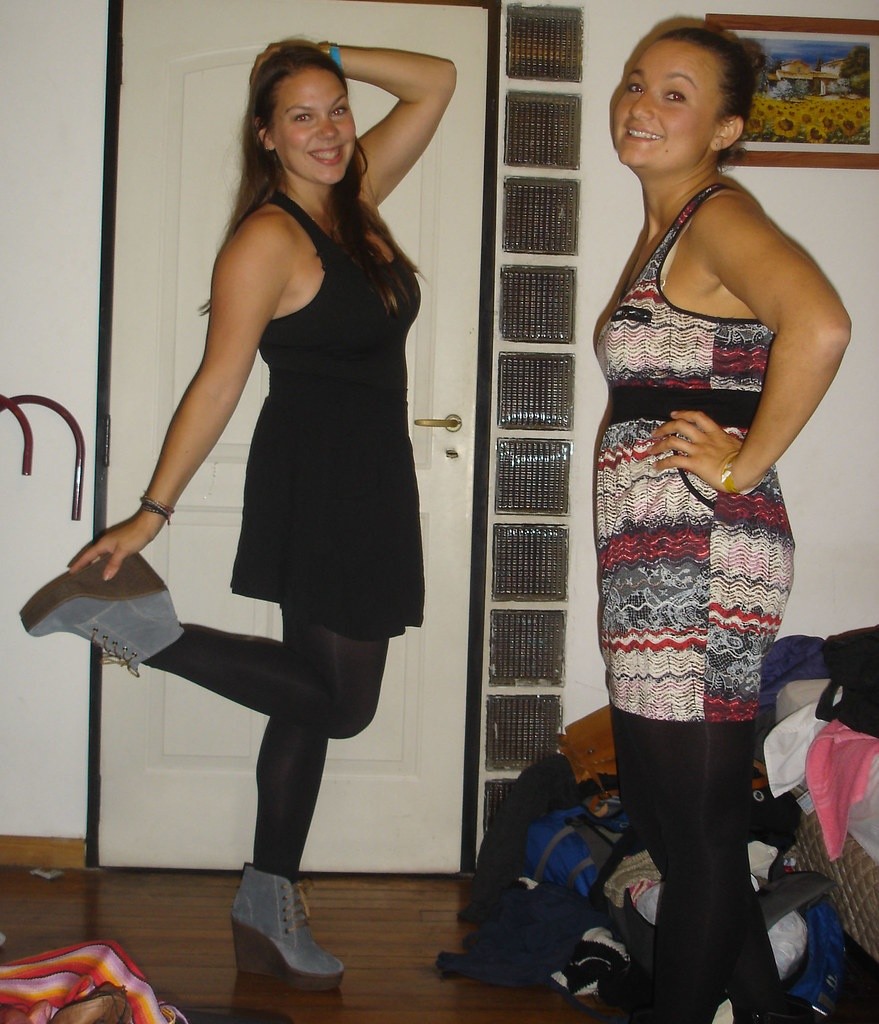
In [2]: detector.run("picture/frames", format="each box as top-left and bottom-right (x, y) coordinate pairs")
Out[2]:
(705, 11), (878, 171)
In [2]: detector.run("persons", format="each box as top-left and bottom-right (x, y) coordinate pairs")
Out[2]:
(585, 24), (854, 1023)
(18, 36), (462, 983)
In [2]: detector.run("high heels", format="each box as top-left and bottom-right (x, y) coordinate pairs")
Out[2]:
(229, 864), (343, 991)
(18, 552), (185, 671)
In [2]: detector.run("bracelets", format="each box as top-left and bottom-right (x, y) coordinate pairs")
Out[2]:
(140, 491), (177, 526)
(720, 456), (766, 497)
